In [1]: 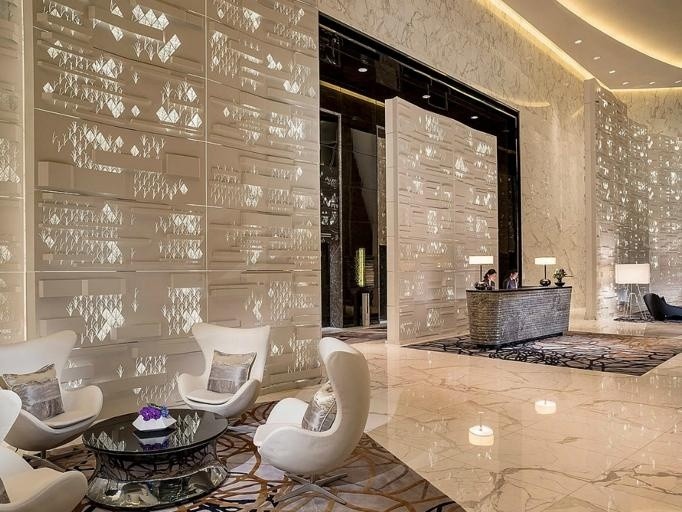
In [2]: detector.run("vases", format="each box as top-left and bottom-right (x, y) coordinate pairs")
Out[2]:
(132, 415), (177, 431)
(132, 433), (172, 445)
(554, 283), (565, 287)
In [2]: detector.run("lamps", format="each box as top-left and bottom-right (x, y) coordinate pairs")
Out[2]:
(468, 255), (493, 290)
(535, 257), (556, 286)
(468, 412), (494, 446)
(534, 395), (557, 415)
(614, 263), (650, 322)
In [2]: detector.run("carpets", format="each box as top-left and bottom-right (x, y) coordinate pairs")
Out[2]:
(402, 329), (682, 377)
(613, 310), (654, 323)
(0, 400), (467, 511)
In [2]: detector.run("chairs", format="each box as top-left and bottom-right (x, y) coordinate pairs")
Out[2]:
(252, 337), (372, 504)
(177, 322), (272, 419)
(0, 330), (104, 511)
(643, 293), (682, 320)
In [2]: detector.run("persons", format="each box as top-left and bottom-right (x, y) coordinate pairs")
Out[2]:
(483, 269), (497, 287)
(503, 269), (519, 288)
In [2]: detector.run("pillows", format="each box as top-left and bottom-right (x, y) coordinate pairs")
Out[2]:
(207, 349), (258, 395)
(302, 382), (337, 433)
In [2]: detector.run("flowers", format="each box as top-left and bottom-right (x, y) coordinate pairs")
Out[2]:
(139, 441), (169, 451)
(138, 403), (170, 421)
(553, 268), (573, 283)
